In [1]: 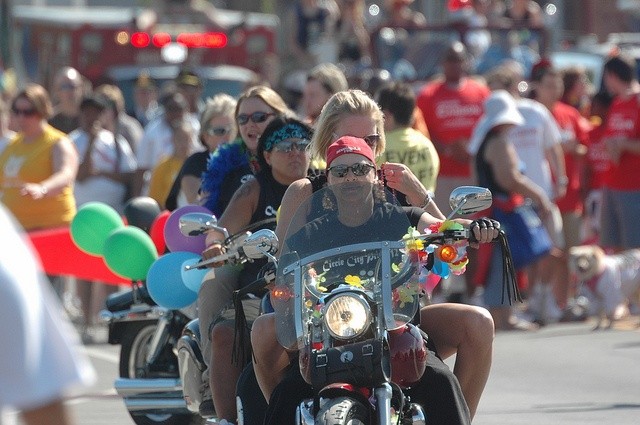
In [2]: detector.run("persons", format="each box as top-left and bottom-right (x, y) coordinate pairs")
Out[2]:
(259, 0), (555, 82)
(175, 73), (202, 114)
(199, 86), (299, 217)
(251, 136), (501, 425)
(418, 41), (491, 302)
(163, 93), (239, 254)
(128, 73), (164, 126)
(47, 68), (84, 134)
(0, 83), (79, 229)
(150, 115), (196, 210)
(304, 63), (348, 124)
(560, 66), (591, 121)
(527, 59), (595, 323)
(132, 89), (200, 198)
(0, 73), (16, 154)
(199, 116), (327, 418)
(377, 83), (440, 198)
(0, 204), (97, 425)
(68, 94), (138, 343)
(487, 60), (586, 322)
(465, 89), (563, 330)
(367, 70), (431, 140)
(208, 90), (446, 424)
(591, 77), (610, 125)
(596, 56), (640, 256)
(92, 84), (145, 154)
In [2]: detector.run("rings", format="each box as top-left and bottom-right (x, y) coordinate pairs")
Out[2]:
(388, 169), (394, 176)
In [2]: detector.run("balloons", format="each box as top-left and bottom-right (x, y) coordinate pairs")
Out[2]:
(125, 197), (160, 235)
(147, 251), (210, 309)
(69, 197), (123, 255)
(164, 204), (217, 253)
(150, 210), (170, 256)
(102, 225), (159, 281)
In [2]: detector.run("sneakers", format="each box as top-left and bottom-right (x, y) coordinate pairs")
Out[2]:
(199, 398), (218, 420)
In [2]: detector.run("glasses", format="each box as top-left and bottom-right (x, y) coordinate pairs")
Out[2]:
(331, 130), (380, 145)
(237, 111), (275, 125)
(272, 140), (310, 153)
(11, 106), (36, 114)
(326, 162), (375, 177)
(207, 127), (232, 137)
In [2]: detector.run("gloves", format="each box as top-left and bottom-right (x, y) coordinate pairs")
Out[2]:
(469, 216), (501, 240)
(257, 261), (278, 280)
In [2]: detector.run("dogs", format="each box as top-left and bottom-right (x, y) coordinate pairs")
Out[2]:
(568, 244), (640, 331)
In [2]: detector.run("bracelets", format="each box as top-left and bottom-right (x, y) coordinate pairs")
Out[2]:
(207, 239), (222, 248)
(419, 193), (431, 210)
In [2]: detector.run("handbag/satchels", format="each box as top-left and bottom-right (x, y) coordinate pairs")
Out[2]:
(504, 205), (554, 266)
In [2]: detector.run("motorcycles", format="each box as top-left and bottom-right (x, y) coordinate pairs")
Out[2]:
(99, 195), (191, 425)
(178, 206), (278, 425)
(233, 185), (506, 425)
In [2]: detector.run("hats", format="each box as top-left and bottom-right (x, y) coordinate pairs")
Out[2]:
(466, 89), (525, 157)
(327, 135), (377, 169)
